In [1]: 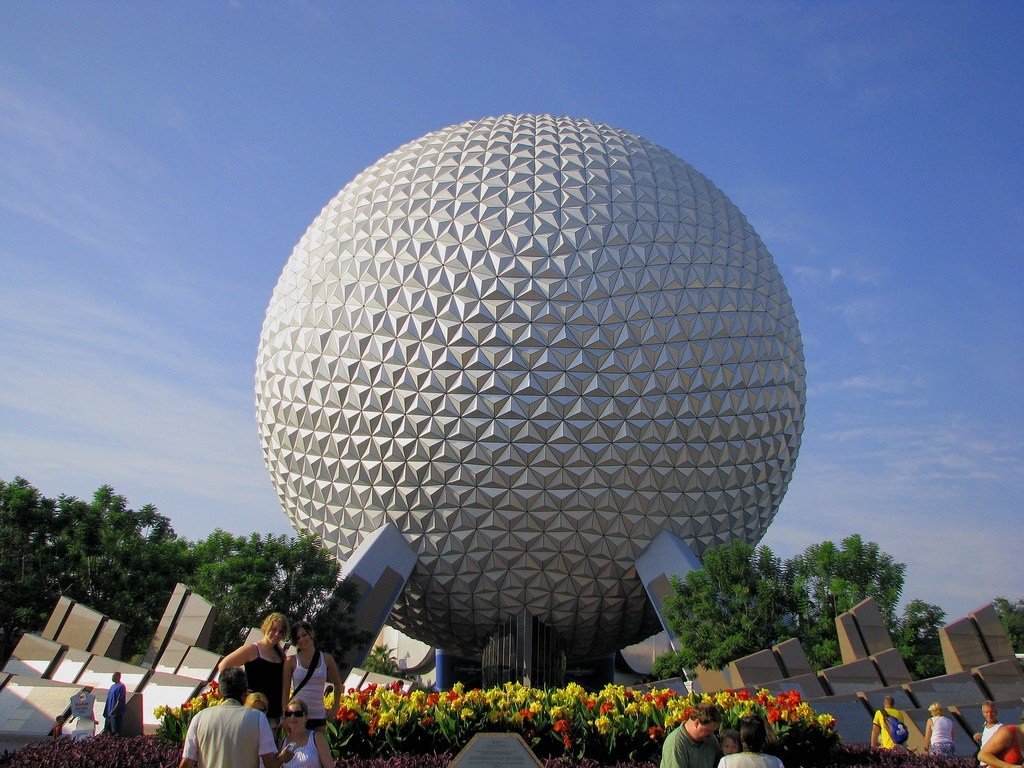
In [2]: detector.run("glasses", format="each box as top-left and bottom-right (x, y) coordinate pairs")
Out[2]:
(258, 708), (266, 713)
(285, 710), (304, 717)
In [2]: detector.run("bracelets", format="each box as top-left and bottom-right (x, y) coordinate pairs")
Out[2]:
(283, 718), (287, 719)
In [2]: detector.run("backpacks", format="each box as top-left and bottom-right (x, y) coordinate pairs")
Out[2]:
(880, 708), (909, 743)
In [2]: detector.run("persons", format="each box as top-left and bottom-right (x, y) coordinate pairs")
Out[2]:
(282, 622), (342, 735)
(871, 696), (908, 753)
(279, 698), (334, 768)
(714, 729), (740, 768)
(180, 666), (295, 768)
(219, 614), (290, 749)
(103, 672), (126, 736)
(973, 702), (1024, 768)
(245, 692), (269, 768)
(925, 704), (956, 756)
(397, 680), (404, 688)
(54, 716), (64, 738)
(660, 703), (721, 768)
(717, 715), (785, 768)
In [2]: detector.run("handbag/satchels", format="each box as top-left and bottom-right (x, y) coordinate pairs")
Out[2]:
(972, 723), (987, 764)
